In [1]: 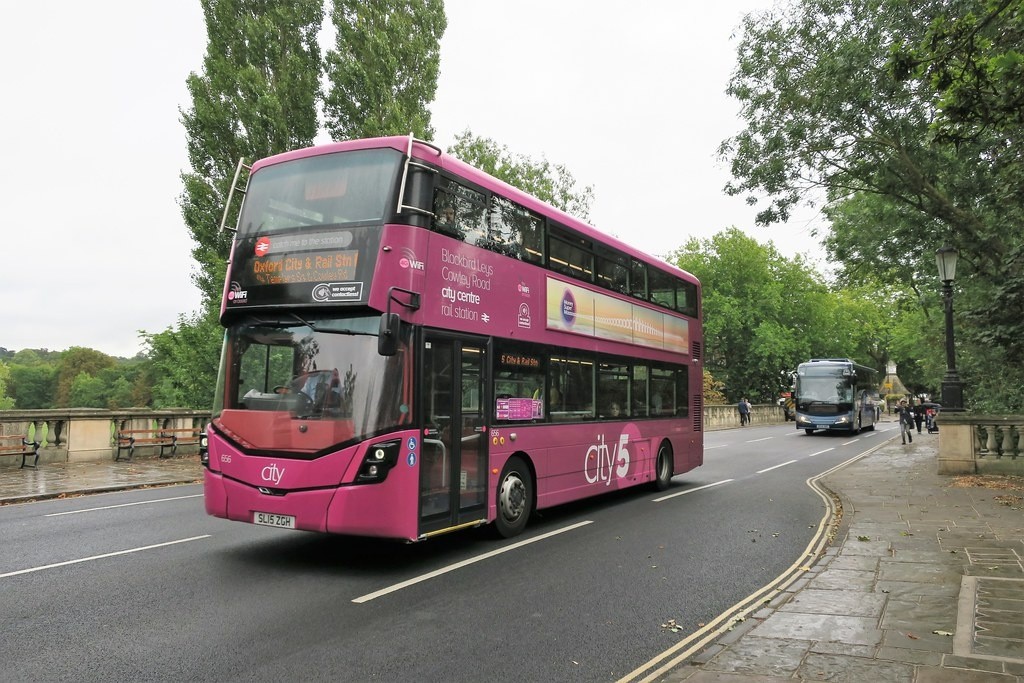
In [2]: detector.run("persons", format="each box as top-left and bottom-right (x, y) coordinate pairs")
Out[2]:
(894, 400), (923, 445)
(279, 353), (345, 405)
(634, 378), (663, 416)
(738, 398), (752, 426)
(533, 368), (585, 411)
(604, 402), (628, 417)
(435, 201), (457, 228)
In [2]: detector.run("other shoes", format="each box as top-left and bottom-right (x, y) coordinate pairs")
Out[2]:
(902, 440), (906, 444)
(918, 431), (921, 434)
(909, 436), (912, 443)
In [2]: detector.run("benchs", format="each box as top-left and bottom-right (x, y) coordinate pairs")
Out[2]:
(116, 428), (201, 460)
(0, 434), (41, 471)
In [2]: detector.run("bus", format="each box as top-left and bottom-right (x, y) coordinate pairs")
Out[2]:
(788, 358), (880, 435)
(203, 132), (705, 546)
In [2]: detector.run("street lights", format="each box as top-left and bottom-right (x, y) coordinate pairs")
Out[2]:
(934, 238), (969, 412)
